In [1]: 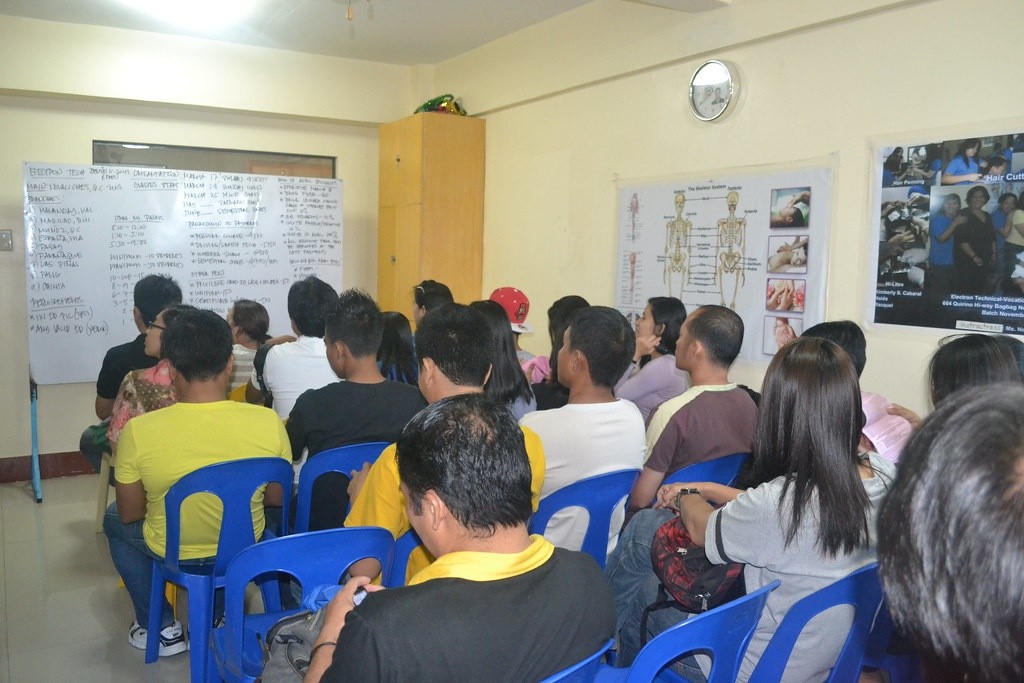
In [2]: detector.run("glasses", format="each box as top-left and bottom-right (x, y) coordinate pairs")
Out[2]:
(147, 320), (166, 332)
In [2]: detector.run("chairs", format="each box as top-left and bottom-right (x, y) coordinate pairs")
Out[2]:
(93, 383), (925, 683)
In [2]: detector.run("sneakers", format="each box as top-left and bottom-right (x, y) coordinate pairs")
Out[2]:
(128, 618), (188, 658)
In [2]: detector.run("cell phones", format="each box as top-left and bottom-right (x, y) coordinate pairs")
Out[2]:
(352, 586), (369, 606)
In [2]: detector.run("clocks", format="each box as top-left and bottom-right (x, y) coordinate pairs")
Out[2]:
(689, 58), (740, 123)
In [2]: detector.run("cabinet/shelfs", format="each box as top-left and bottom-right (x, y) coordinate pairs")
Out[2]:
(377, 111), (485, 334)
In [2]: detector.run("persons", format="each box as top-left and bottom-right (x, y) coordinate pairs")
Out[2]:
(767, 280), (797, 349)
(517, 306), (646, 558)
(768, 235), (809, 271)
(625, 304), (759, 513)
(519, 296), (588, 411)
(928, 334), (1024, 407)
(878, 134), (1024, 297)
(606, 339), (895, 683)
(875, 379), (1024, 683)
(80, 275), (546, 655)
(770, 191), (810, 227)
(614, 297), (688, 425)
(800, 320), (866, 379)
(305, 393), (619, 683)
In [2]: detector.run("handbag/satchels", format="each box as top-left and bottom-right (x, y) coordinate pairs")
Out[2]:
(650, 513), (745, 621)
(254, 601), (336, 682)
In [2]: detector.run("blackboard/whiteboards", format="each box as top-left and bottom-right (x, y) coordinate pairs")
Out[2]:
(21, 160), (342, 385)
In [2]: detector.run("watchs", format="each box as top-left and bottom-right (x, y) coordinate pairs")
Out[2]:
(676, 487), (700, 506)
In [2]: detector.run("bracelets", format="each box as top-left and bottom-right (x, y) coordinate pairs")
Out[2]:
(632, 360), (636, 364)
(308, 642), (336, 664)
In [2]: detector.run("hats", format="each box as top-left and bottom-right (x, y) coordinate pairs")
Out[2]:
(488, 286), (535, 335)
